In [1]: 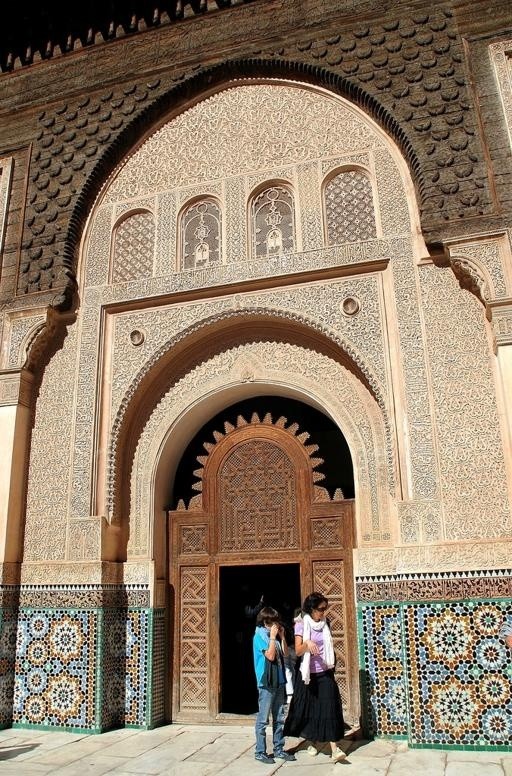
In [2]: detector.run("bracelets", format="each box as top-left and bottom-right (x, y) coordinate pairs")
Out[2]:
(269, 638), (275, 641)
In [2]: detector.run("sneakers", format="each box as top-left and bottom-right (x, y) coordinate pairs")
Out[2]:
(308, 744), (317, 755)
(275, 750), (294, 761)
(332, 748), (346, 760)
(255, 753), (274, 763)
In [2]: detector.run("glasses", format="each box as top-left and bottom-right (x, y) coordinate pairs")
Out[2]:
(315, 608), (326, 611)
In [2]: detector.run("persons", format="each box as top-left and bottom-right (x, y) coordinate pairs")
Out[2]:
(253, 605), (296, 765)
(282, 592), (348, 764)
